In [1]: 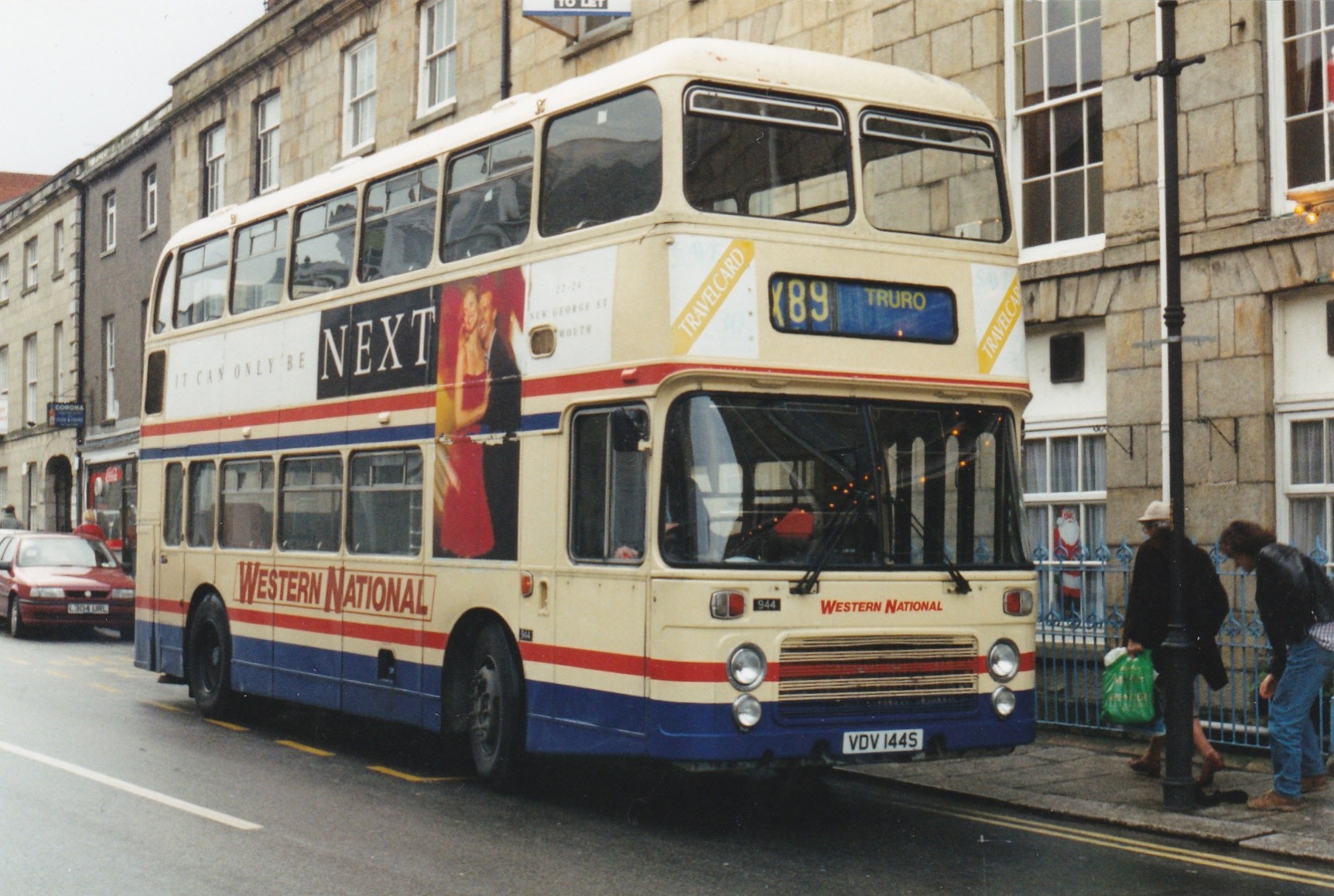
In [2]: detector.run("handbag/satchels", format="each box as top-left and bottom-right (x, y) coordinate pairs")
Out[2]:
(1100, 646), (1159, 725)
(1309, 622), (1334, 652)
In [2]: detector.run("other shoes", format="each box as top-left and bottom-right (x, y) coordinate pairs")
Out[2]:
(1196, 752), (1225, 786)
(1247, 788), (1300, 809)
(1128, 738), (1160, 776)
(1299, 775), (1323, 790)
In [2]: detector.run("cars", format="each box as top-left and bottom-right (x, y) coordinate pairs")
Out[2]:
(0, 533), (136, 641)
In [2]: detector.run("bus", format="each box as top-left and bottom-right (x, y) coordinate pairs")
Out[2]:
(133, 34), (1039, 798)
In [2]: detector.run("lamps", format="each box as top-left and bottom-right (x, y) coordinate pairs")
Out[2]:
(1294, 199), (1321, 225)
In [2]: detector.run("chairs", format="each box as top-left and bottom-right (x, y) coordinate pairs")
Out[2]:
(189, 502), (363, 552)
(658, 475), (711, 557)
(205, 220), (604, 321)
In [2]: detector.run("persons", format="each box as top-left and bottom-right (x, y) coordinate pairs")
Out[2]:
(1052, 507), (1088, 622)
(72, 510), (106, 542)
(609, 436), (713, 566)
(98, 507), (136, 579)
(1216, 520), (1334, 808)
(1121, 500), (1231, 785)
(444, 281), (517, 561)
(0, 506), (23, 529)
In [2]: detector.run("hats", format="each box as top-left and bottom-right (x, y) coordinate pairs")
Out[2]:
(1137, 500), (1169, 520)
